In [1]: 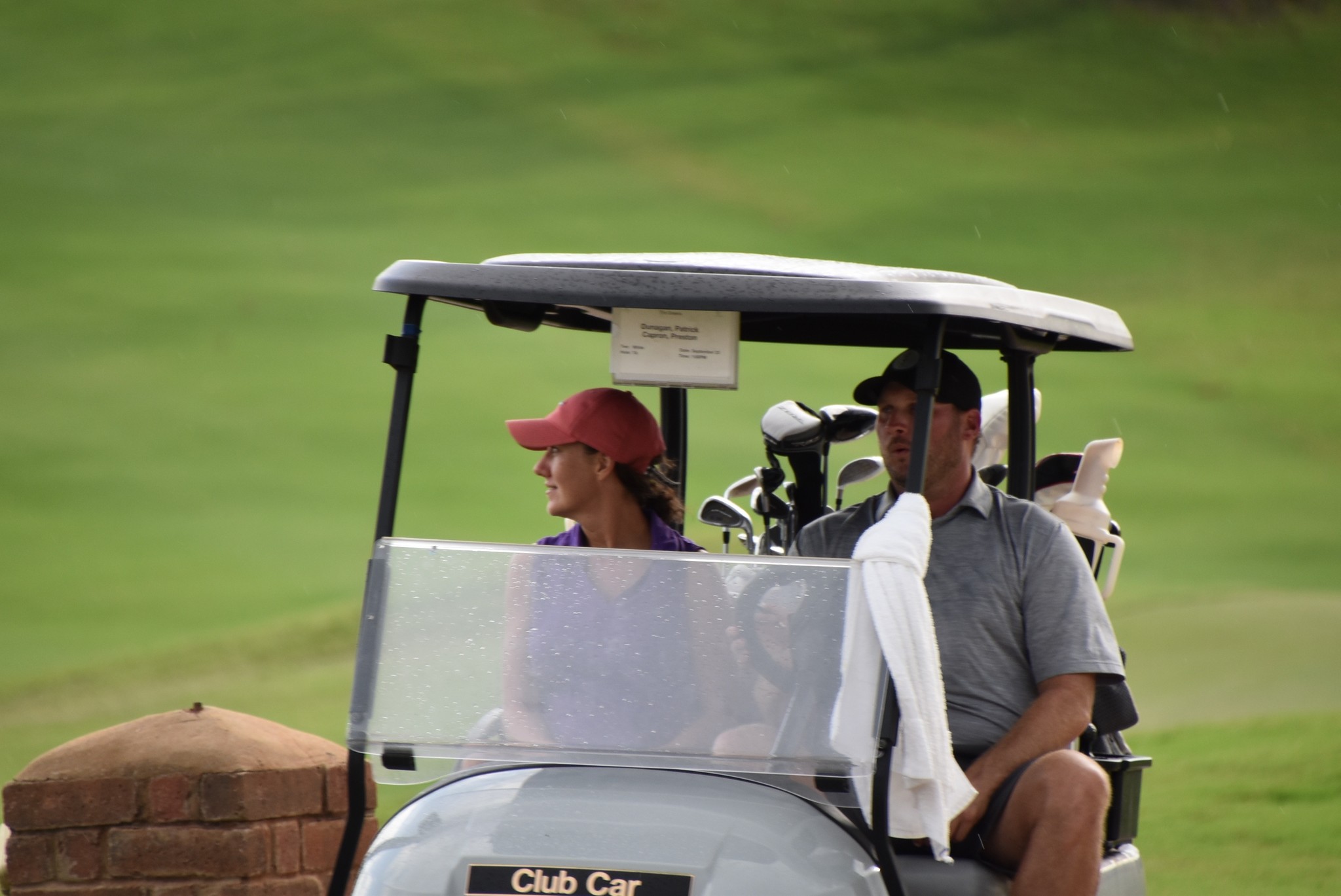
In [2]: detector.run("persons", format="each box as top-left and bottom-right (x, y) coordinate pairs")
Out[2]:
(504, 387), (738, 756)
(714, 347), (1124, 896)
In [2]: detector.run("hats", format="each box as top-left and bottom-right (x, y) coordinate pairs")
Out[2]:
(854, 346), (981, 411)
(505, 387), (668, 475)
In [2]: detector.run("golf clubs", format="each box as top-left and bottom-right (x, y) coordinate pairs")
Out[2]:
(697, 454), (886, 561)
